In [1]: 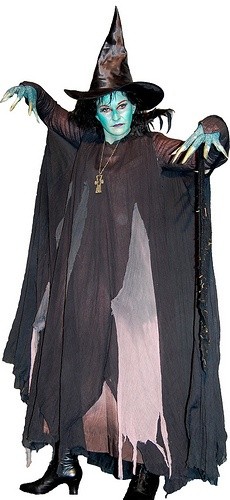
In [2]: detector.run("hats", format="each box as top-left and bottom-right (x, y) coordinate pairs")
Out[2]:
(63, 6), (164, 111)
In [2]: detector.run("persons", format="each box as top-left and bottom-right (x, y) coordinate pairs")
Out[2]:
(0, 74), (230, 500)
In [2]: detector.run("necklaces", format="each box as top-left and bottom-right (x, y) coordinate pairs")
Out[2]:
(95, 133), (126, 194)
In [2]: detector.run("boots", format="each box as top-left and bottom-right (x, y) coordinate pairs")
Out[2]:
(122, 462), (160, 500)
(18, 439), (83, 495)
